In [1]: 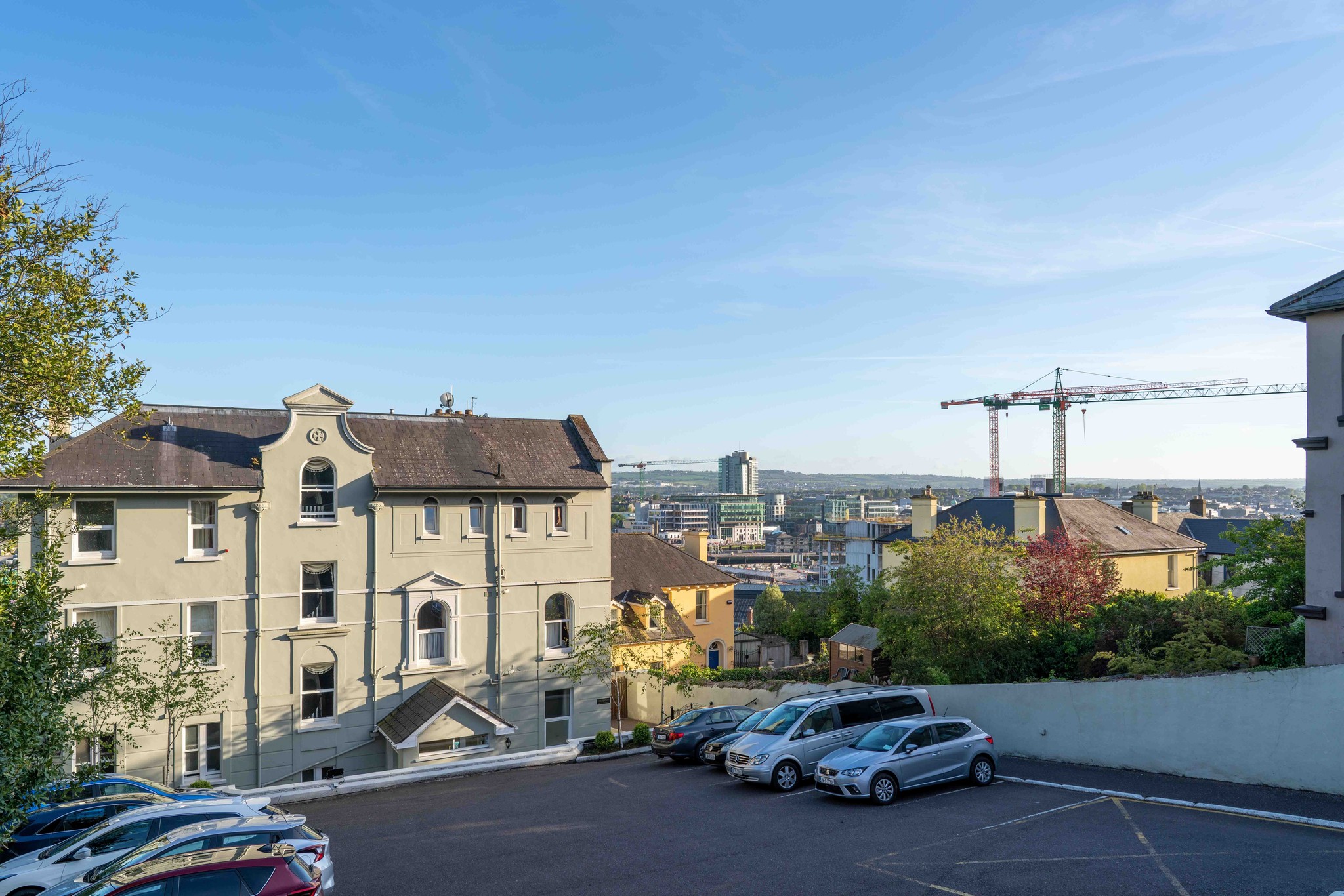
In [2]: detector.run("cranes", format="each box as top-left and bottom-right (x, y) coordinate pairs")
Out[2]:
(619, 459), (729, 503)
(940, 366), (1307, 496)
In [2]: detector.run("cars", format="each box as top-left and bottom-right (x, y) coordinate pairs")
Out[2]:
(813, 716), (1000, 806)
(650, 704), (805, 770)
(0, 773), (336, 895)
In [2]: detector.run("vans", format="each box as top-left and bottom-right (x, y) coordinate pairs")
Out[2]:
(724, 684), (935, 792)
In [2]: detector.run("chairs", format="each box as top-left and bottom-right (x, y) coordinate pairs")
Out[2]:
(842, 669), (857, 680)
(920, 731), (935, 747)
(831, 667), (848, 681)
(820, 720), (832, 732)
(898, 729), (915, 750)
(789, 715), (815, 739)
(719, 711), (728, 721)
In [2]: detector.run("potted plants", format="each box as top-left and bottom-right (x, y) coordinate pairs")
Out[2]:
(766, 658), (775, 669)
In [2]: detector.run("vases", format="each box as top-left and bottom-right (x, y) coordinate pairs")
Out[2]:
(807, 656), (814, 663)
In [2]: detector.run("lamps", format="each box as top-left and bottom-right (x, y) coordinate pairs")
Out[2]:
(729, 646), (734, 652)
(505, 737), (511, 748)
(325, 768), (344, 781)
(702, 649), (706, 655)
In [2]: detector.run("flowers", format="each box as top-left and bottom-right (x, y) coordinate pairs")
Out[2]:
(806, 653), (816, 656)
